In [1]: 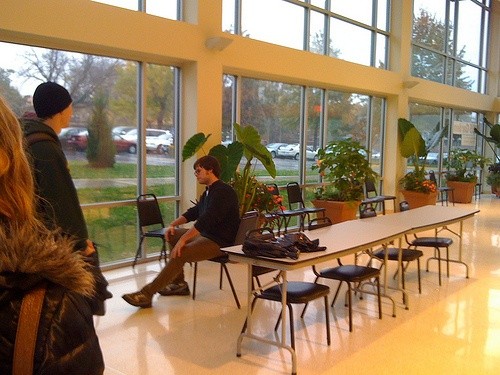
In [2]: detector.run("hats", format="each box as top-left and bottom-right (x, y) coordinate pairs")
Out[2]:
(33, 81), (72, 118)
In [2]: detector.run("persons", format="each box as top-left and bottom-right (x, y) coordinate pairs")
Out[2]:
(18, 82), (112, 327)
(120, 156), (243, 307)
(1, 97), (106, 375)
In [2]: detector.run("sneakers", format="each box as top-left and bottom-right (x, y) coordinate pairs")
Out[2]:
(122, 292), (152, 308)
(158, 281), (190, 296)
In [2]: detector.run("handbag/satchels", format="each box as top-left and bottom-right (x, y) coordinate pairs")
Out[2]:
(283, 233), (327, 252)
(242, 233), (300, 261)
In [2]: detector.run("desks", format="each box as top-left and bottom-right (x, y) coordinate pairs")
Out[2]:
(220, 206), (480, 375)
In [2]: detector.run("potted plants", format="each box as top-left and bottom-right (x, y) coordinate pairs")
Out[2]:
(445, 141), (490, 202)
(311, 137), (376, 228)
(184, 122), (276, 255)
(397, 117), (447, 209)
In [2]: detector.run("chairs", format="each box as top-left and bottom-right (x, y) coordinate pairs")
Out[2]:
(133, 170), (453, 349)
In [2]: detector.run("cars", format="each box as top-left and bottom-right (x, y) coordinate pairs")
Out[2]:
(277, 142), (318, 162)
(307, 144), (381, 161)
(418, 152), (448, 166)
(264, 143), (288, 159)
(57, 126), (174, 155)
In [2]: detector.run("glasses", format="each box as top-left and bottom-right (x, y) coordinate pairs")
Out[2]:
(194, 168), (207, 175)
